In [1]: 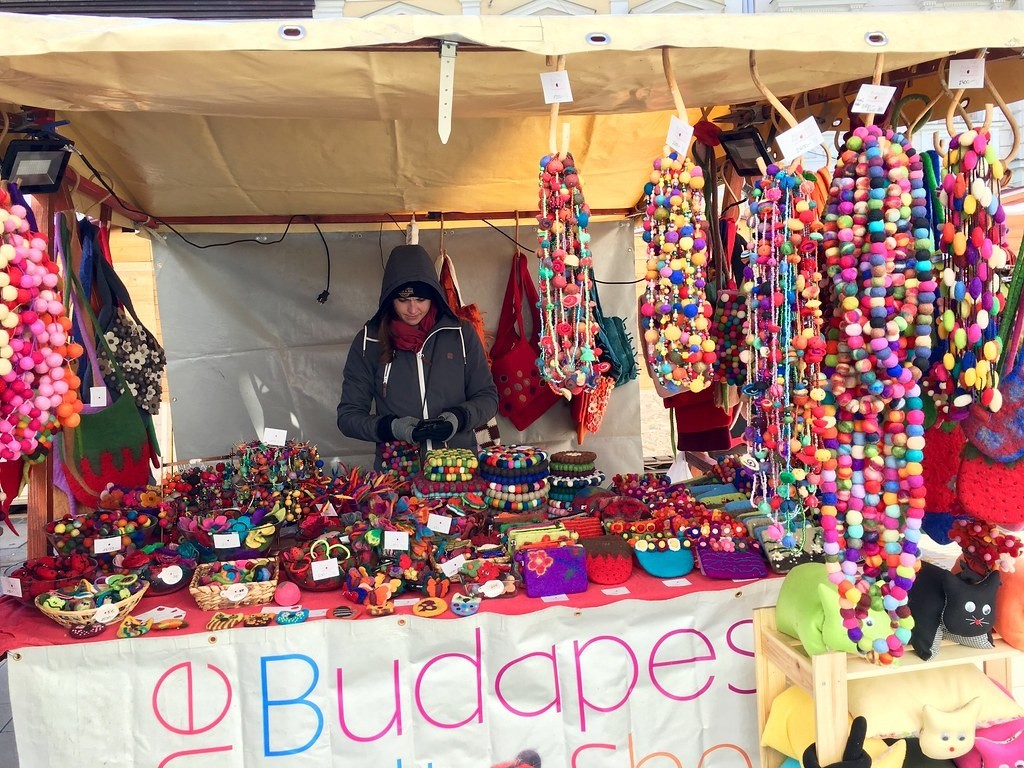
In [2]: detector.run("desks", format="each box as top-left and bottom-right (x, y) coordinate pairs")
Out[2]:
(6, 476), (796, 766)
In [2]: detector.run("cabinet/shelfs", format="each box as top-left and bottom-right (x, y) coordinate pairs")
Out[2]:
(746, 606), (1024, 768)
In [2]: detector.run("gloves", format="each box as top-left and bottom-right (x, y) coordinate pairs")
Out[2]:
(392, 416), (428, 445)
(429, 412), (459, 443)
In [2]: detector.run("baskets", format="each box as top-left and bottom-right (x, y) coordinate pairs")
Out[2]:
(34, 580), (150, 630)
(189, 556), (279, 611)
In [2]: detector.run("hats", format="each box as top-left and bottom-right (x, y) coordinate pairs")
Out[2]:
(386, 281), (435, 300)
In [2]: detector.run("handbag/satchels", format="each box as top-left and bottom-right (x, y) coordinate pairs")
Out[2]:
(434, 254), (494, 367)
(490, 253), (562, 431)
(48, 211), (167, 516)
(588, 265), (641, 388)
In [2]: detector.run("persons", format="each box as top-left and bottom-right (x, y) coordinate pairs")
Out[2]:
(336, 244), (501, 471)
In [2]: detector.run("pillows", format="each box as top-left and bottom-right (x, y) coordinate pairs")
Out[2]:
(847, 660), (1023, 760)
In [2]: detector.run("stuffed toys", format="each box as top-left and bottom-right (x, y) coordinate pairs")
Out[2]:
(760, 557), (1023, 768)
